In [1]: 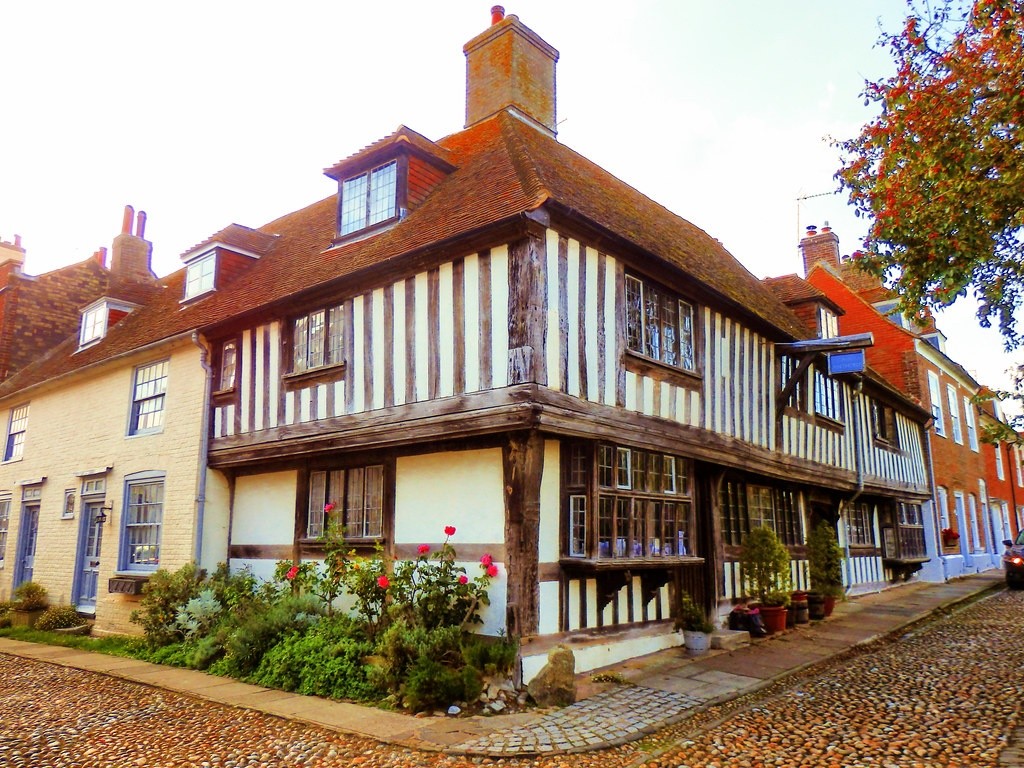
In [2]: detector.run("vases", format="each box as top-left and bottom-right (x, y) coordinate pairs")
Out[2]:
(945, 538), (956, 546)
(787, 592), (825, 624)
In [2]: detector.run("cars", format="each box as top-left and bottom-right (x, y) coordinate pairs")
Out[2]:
(1002, 528), (1024, 591)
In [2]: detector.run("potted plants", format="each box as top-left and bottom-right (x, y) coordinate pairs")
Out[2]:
(671, 587), (717, 657)
(739, 526), (794, 632)
(803, 519), (845, 618)
(8, 581), (48, 627)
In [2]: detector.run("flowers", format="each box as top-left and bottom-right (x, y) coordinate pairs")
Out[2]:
(941, 527), (961, 543)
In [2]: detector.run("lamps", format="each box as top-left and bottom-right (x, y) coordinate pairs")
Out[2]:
(95, 508), (112, 523)
